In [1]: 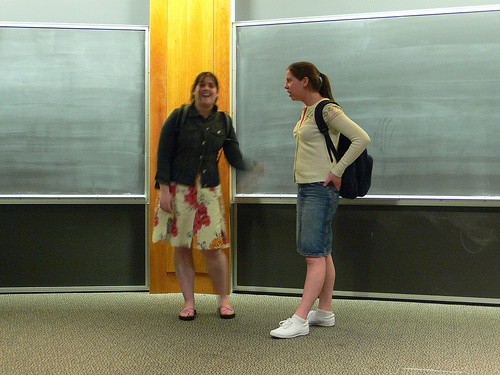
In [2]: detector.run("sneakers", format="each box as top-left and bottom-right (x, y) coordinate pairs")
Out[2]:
(270, 317), (309, 338)
(307, 310), (335, 326)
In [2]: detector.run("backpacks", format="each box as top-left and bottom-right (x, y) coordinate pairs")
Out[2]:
(314, 100), (373, 199)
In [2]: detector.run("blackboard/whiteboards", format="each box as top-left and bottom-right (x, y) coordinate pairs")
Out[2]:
(0, 21), (151, 204)
(229, 4), (500, 207)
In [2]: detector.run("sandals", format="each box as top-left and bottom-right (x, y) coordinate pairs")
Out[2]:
(179, 305), (196, 321)
(219, 301), (235, 319)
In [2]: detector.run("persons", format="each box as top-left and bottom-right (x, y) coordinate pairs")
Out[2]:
(270, 62), (371, 339)
(151, 72), (265, 320)
